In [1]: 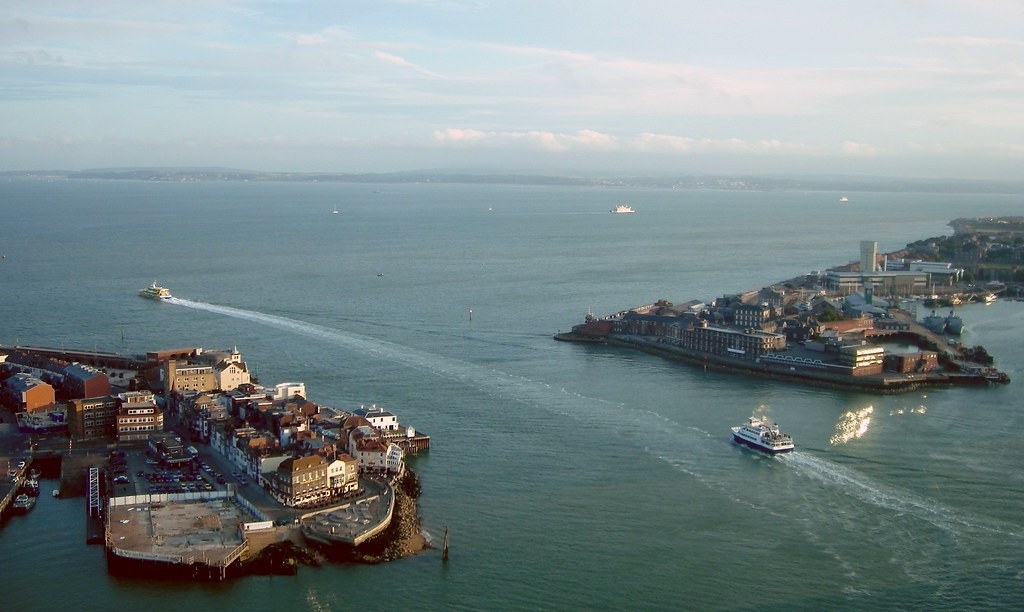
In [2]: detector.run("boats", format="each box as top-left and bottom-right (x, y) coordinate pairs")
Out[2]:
(609, 203), (636, 213)
(921, 279), (999, 337)
(137, 280), (172, 300)
(730, 415), (795, 455)
(12, 468), (40, 516)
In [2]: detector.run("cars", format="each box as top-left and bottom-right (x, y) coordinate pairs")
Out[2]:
(9, 470), (17, 477)
(110, 448), (249, 496)
(17, 461), (26, 468)
